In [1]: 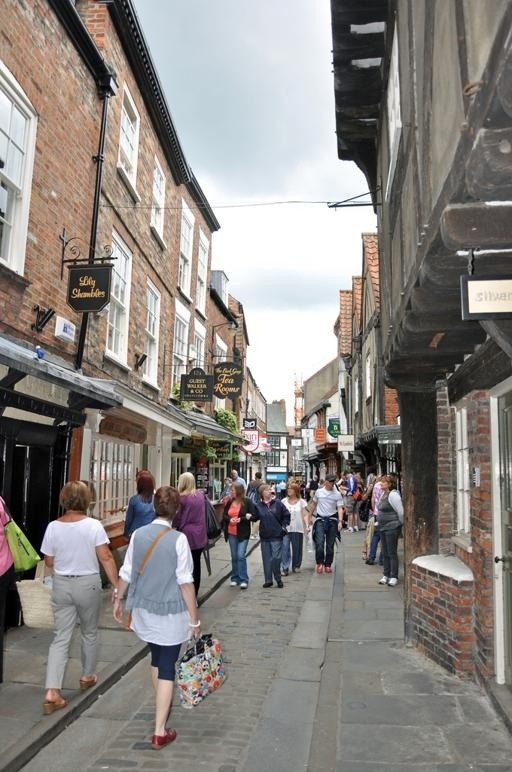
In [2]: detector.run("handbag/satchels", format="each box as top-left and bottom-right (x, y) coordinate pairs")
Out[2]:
(125, 527), (175, 633)
(266, 504), (288, 536)
(203, 494), (221, 540)
(175, 632), (228, 710)
(16, 560), (64, 629)
(0, 500), (42, 574)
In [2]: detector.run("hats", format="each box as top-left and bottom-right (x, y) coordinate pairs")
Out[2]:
(325, 473), (337, 482)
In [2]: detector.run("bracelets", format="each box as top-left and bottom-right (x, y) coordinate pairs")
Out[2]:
(189, 619), (201, 628)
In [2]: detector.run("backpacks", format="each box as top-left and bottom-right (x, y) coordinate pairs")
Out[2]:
(247, 480), (263, 505)
(359, 491), (373, 523)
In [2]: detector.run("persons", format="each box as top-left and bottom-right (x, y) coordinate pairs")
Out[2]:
(270, 483), (276, 500)
(334, 472), (340, 490)
(309, 475), (318, 499)
(0, 495), (15, 685)
(280, 480), (286, 498)
(246, 472), (265, 540)
(40, 481), (119, 715)
(123, 472), (155, 543)
(353, 480), (366, 530)
(337, 471), (348, 528)
(226, 470), (247, 498)
(308, 474), (344, 574)
(223, 481), (255, 588)
(112, 485), (201, 751)
(378, 475), (404, 587)
(280, 483), (311, 576)
(299, 481), (305, 499)
(275, 482), (281, 499)
(317, 479), (325, 489)
(172, 472), (208, 608)
(365, 476), (385, 565)
(217, 478), (232, 503)
(362, 473), (376, 560)
(340, 470), (359, 533)
(306, 479), (312, 501)
(245, 484), (291, 588)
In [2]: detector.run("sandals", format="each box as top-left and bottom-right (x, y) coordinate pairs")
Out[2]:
(79, 674), (98, 690)
(43, 698), (69, 715)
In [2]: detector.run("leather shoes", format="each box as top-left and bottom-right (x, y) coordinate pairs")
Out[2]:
(150, 728), (177, 749)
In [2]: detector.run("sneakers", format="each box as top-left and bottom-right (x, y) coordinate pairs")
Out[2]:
(317, 565), (322, 574)
(326, 566), (332, 573)
(389, 578), (399, 586)
(378, 576), (389, 585)
(241, 582), (248, 589)
(230, 581), (238, 587)
(278, 581), (284, 589)
(263, 582), (274, 588)
